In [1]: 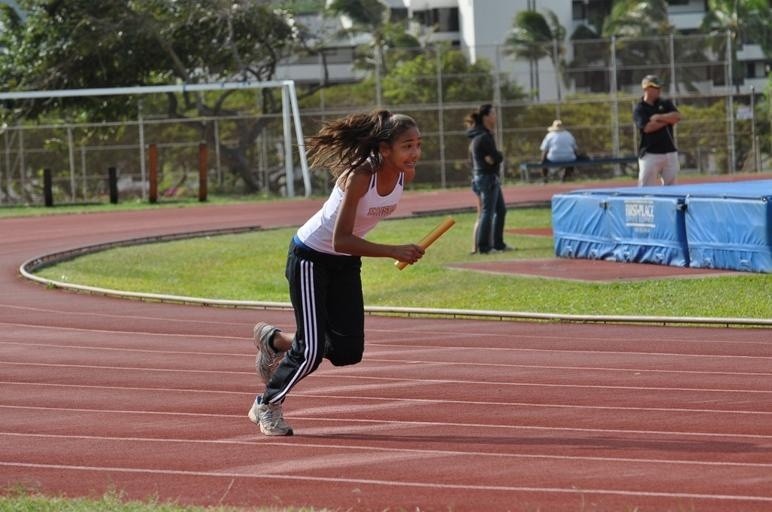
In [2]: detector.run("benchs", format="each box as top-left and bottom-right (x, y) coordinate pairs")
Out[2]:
(520, 156), (638, 183)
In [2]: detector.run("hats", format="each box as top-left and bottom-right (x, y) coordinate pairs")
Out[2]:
(642, 75), (664, 90)
(545, 119), (567, 132)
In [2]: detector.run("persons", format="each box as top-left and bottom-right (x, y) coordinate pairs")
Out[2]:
(631, 74), (683, 188)
(464, 111), (479, 253)
(541, 119), (578, 183)
(465, 104), (521, 255)
(246, 108), (422, 437)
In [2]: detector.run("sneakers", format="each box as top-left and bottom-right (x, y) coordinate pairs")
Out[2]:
(473, 243), (520, 256)
(252, 321), (287, 386)
(247, 392), (294, 437)
